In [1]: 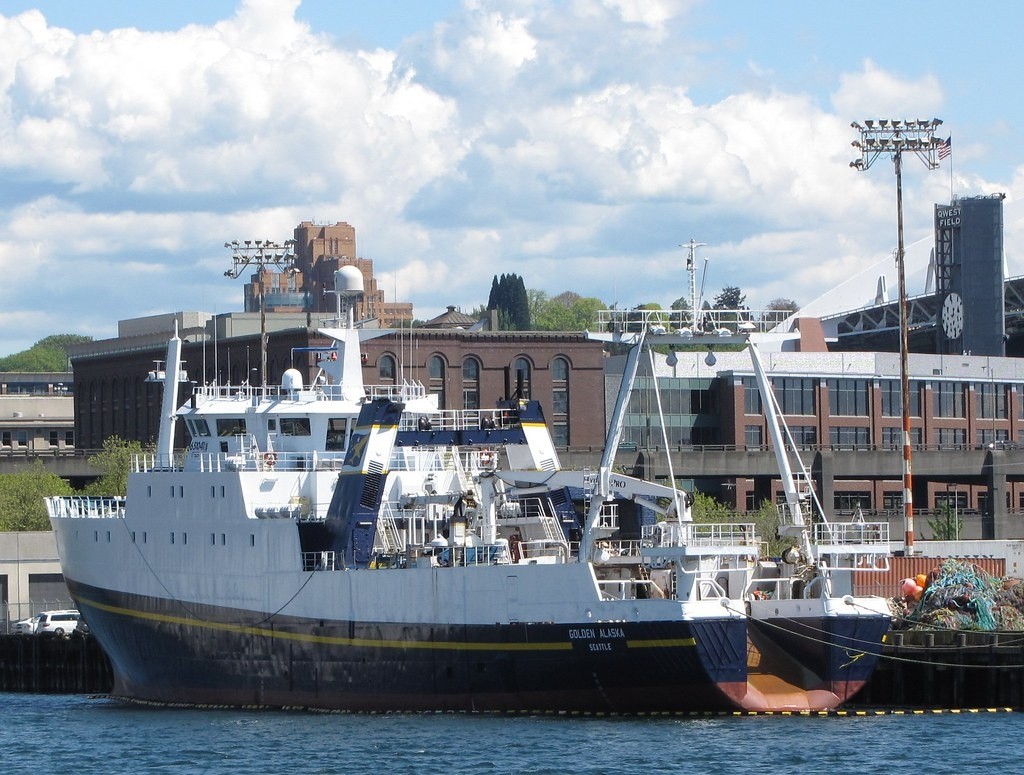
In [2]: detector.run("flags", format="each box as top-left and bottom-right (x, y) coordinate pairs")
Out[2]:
(937, 136), (952, 160)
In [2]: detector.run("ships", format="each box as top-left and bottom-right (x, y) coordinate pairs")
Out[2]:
(37, 263), (896, 716)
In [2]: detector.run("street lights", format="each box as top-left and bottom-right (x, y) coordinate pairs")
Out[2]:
(848, 117), (946, 555)
(224, 239), (299, 397)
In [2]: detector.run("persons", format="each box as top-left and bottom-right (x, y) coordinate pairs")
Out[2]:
(739, 525), (750, 542)
(755, 587), (766, 601)
(418, 412), (508, 431)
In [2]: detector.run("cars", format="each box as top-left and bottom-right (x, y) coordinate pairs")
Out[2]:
(38, 613), (89, 638)
(11, 609), (80, 636)
